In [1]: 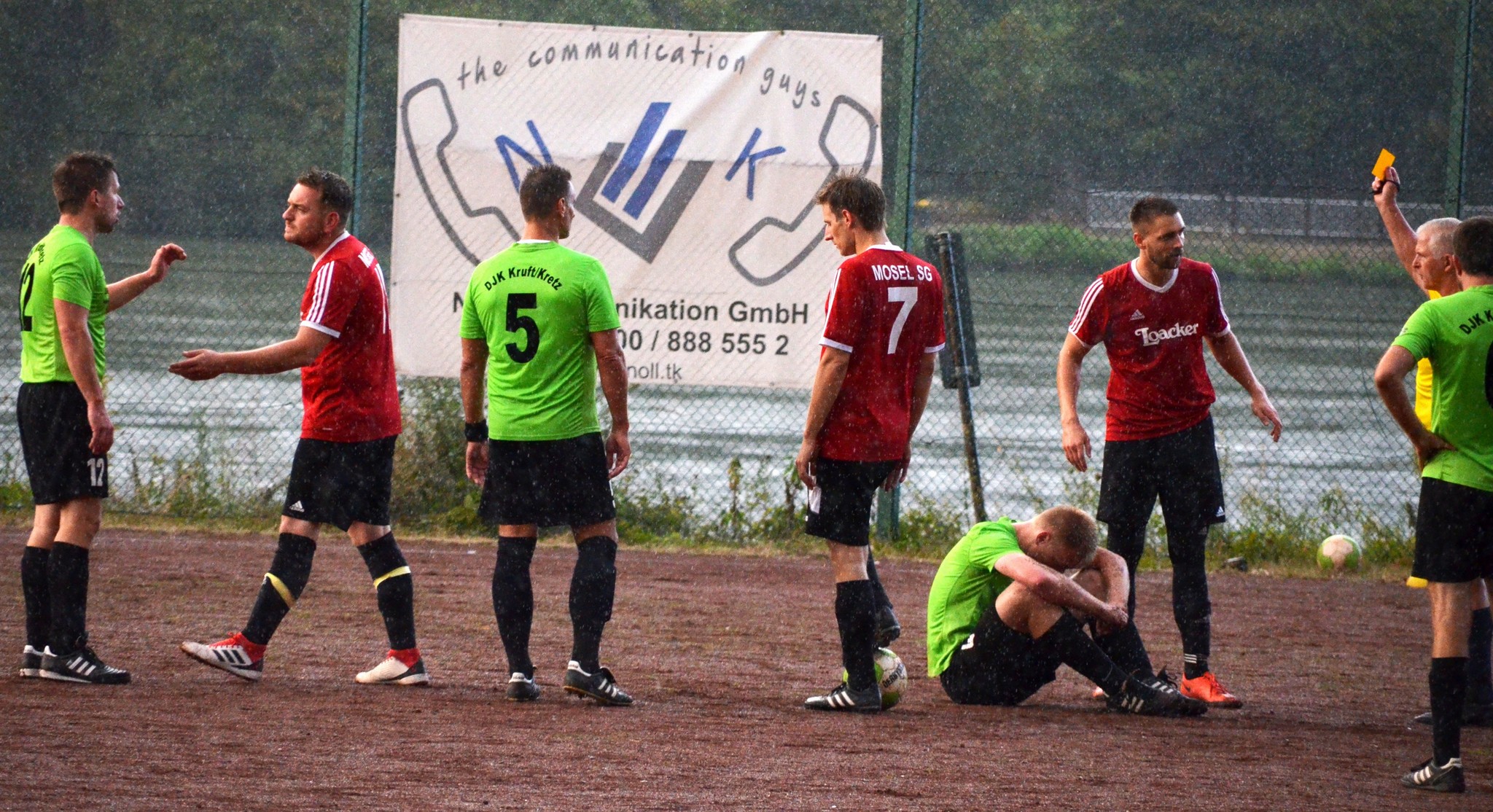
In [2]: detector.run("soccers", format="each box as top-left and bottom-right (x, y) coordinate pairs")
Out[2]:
(842, 647), (907, 710)
(1317, 534), (1360, 571)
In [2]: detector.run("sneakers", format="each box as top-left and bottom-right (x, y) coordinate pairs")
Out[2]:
(563, 660), (633, 705)
(803, 683), (882, 711)
(1106, 669), (1180, 715)
(506, 675), (542, 702)
(1402, 758), (1465, 791)
(1181, 671), (1241, 709)
(179, 632), (268, 681)
(1454, 700), (1493, 727)
(1138, 665), (1208, 716)
(355, 648), (429, 685)
(18, 645), (44, 678)
(871, 606), (900, 652)
(1414, 711), (1432, 724)
(38, 631), (132, 684)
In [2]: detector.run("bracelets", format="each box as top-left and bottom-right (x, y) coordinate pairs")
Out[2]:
(464, 418), (489, 442)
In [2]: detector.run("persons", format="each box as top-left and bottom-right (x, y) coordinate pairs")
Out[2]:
(926, 505), (1208, 717)
(795, 174), (947, 714)
(1372, 215), (1493, 793)
(1055, 197), (1283, 711)
(168, 170), (428, 687)
(16, 151), (187, 684)
(1372, 163), (1493, 727)
(459, 165), (634, 707)
